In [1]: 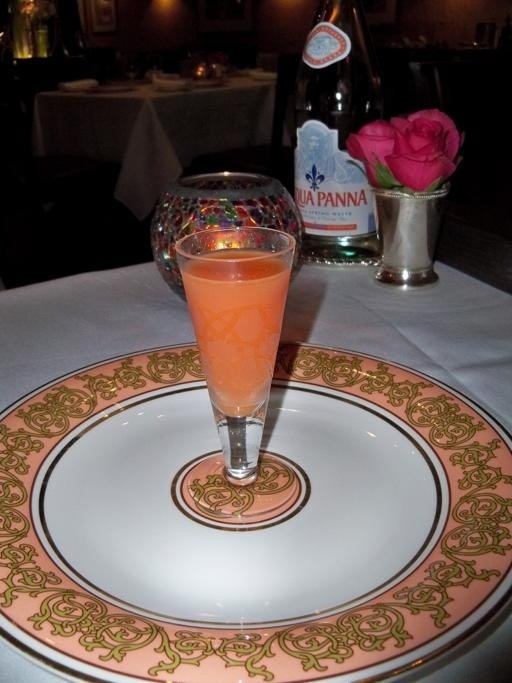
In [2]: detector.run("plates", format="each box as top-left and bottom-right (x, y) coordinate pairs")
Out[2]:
(61, 56), (281, 89)
(1, 336), (511, 682)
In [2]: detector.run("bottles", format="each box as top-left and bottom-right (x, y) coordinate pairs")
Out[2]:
(284, 1), (392, 271)
(149, 169), (305, 303)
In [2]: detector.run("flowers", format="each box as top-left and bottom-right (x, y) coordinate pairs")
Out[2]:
(346, 109), (462, 193)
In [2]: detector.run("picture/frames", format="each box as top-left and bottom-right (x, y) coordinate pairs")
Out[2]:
(85, 0), (118, 35)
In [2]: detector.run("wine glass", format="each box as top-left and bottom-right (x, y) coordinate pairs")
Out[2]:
(167, 228), (318, 530)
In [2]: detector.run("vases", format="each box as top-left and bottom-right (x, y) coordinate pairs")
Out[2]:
(379, 185), (440, 286)
